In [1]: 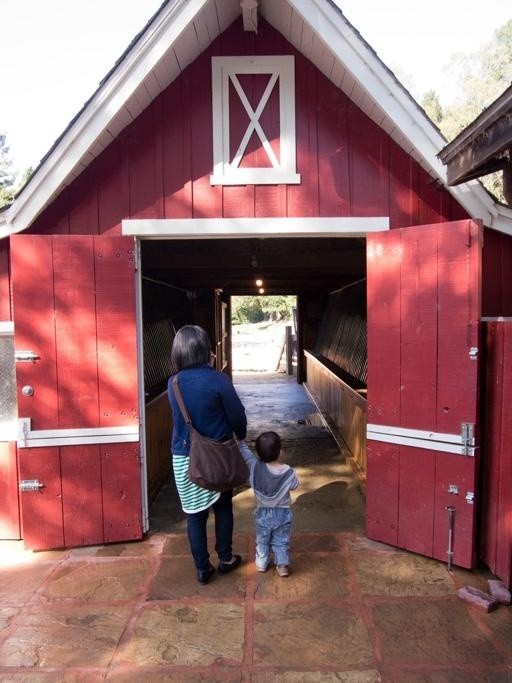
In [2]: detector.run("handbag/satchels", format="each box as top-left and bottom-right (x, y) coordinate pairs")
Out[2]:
(189, 424), (250, 491)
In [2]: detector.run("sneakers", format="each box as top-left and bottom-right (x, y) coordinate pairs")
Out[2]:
(276, 564), (290, 576)
(256, 565), (266, 572)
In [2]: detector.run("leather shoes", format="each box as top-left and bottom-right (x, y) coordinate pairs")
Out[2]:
(198, 565), (214, 584)
(218, 555), (241, 575)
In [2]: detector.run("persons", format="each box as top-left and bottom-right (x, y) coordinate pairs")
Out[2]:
(232, 432), (300, 578)
(165, 324), (247, 585)
